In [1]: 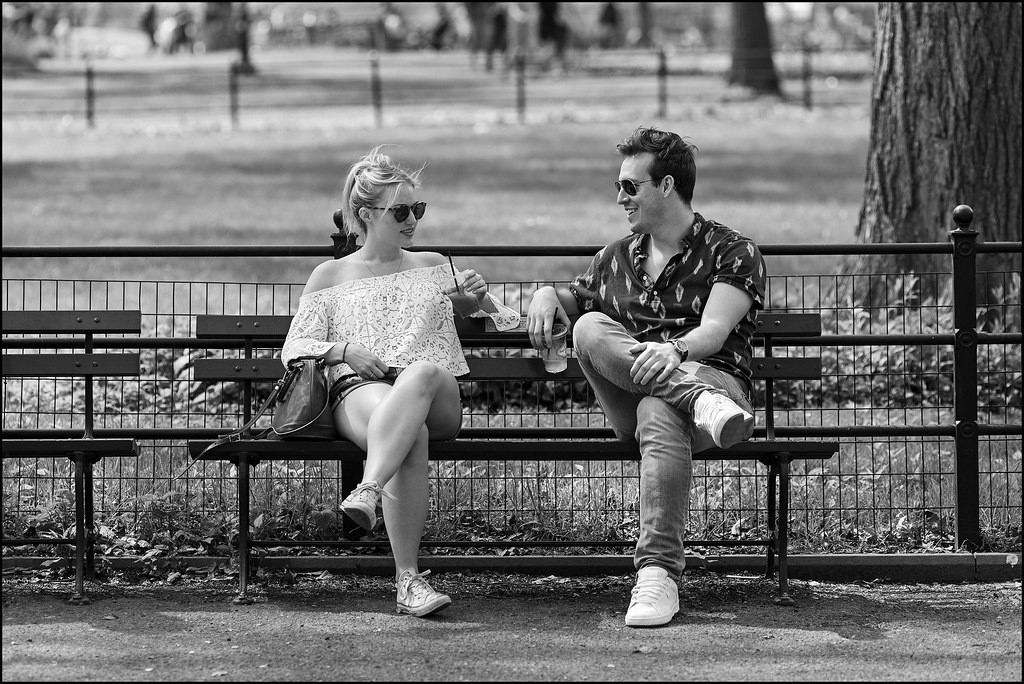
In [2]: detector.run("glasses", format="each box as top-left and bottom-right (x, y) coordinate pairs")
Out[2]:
(356, 201), (427, 223)
(613, 176), (681, 196)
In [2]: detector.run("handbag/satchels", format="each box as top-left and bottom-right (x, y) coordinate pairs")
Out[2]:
(169, 355), (336, 481)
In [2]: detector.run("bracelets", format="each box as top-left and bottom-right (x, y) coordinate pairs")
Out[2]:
(342, 343), (350, 362)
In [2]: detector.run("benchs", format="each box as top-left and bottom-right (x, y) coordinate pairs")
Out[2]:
(0, 309), (141, 604)
(190, 311), (838, 609)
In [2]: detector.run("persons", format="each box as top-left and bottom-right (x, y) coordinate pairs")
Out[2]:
(280, 154), (521, 617)
(526, 129), (767, 626)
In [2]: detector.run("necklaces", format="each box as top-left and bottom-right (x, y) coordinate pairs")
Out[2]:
(360, 249), (403, 276)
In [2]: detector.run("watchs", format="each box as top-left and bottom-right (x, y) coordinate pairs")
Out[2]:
(666, 339), (689, 363)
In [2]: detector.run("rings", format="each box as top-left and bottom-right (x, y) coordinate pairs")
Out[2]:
(370, 374), (374, 378)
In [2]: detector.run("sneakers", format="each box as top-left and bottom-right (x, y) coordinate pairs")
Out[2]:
(694, 389), (755, 449)
(339, 481), (399, 531)
(395, 566), (452, 617)
(624, 566), (680, 626)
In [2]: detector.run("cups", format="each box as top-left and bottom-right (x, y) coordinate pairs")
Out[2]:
(541, 324), (567, 373)
(439, 269), (481, 319)
